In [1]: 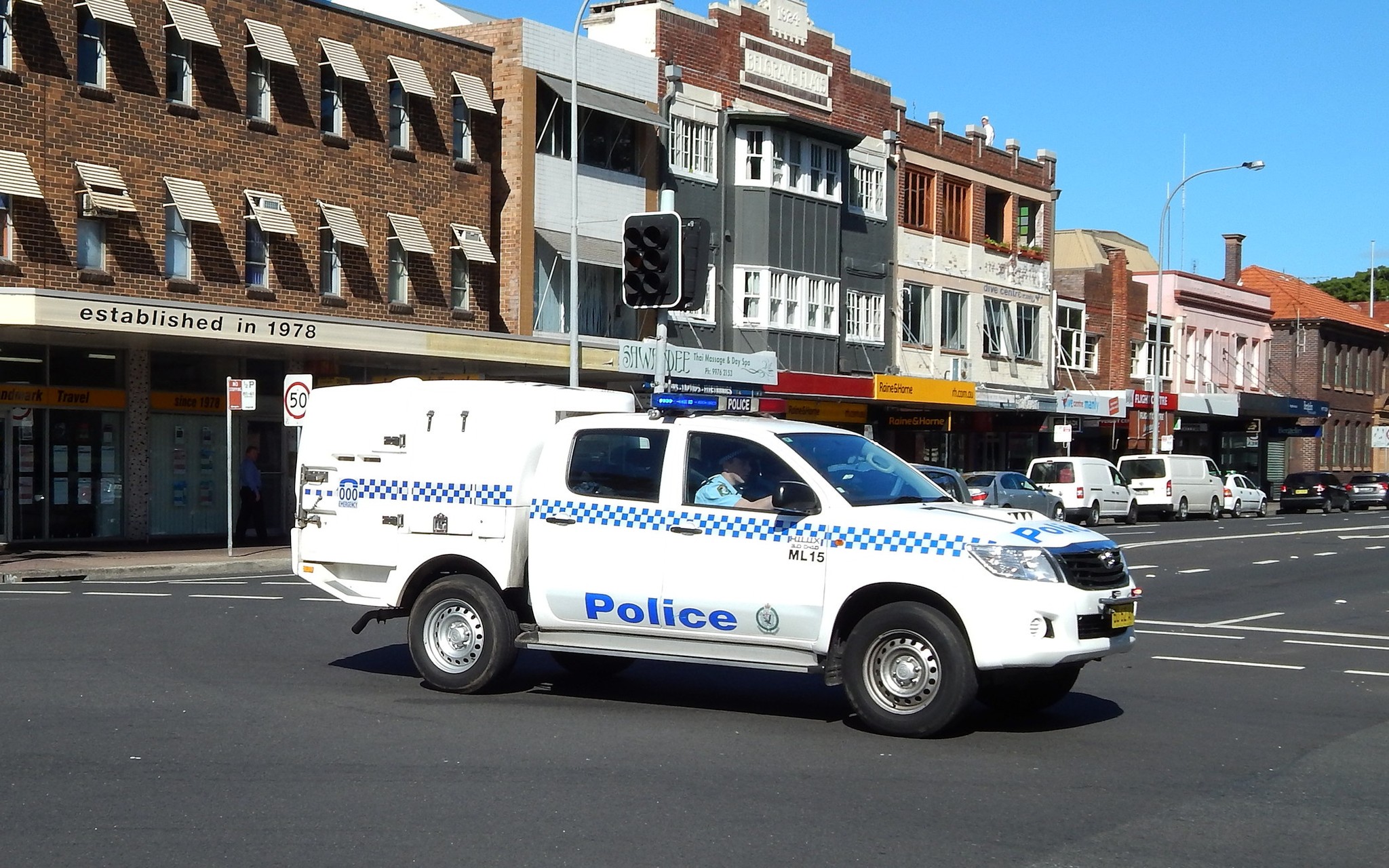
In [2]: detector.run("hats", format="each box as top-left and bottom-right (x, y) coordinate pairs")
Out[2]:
(717, 442), (762, 466)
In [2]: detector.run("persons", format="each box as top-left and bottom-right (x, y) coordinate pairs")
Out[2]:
(235, 446), (268, 545)
(693, 438), (784, 510)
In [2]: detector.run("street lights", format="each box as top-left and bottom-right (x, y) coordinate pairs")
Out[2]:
(1152, 160), (1266, 454)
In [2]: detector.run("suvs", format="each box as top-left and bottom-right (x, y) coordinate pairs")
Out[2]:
(1279, 472), (1350, 514)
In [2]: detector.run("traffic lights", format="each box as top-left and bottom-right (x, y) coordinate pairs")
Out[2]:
(621, 212), (680, 308)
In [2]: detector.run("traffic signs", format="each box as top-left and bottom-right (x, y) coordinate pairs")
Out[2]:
(228, 379), (255, 411)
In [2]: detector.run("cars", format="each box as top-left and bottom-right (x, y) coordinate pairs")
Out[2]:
(908, 463), (1066, 523)
(1213, 473), (1268, 518)
(1344, 473), (1389, 510)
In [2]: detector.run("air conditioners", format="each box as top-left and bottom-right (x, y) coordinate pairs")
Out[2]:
(1143, 376), (1164, 393)
(950, 357), (973, 382)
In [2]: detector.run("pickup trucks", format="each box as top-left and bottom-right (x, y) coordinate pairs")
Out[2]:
(290, 376), (1141, 740)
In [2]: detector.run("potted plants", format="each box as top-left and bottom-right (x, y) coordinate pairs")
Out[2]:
(985, 235), (997, 250)
(1030, 245), (1046, 259)
(1017, 242), (1032, 256)
(998, 241), (1009, 252)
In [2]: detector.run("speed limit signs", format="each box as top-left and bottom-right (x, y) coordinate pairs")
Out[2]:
(283, 375), (312, 427)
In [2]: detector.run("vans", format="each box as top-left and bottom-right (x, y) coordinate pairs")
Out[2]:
(1116, 454), (1228, 522)
(1024, 457), (1138, 526)
